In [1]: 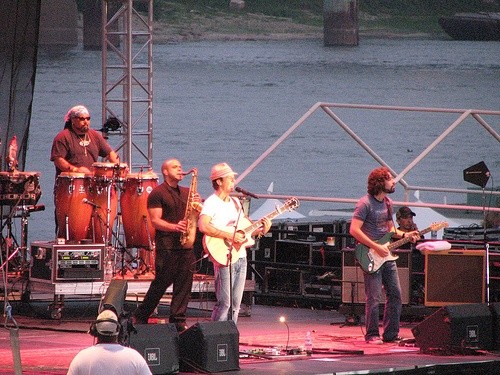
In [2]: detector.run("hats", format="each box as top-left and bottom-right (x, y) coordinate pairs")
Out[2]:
(396, 207), (416, 219)
(95, 309), (119, 337)
(209, 162), (238, 181)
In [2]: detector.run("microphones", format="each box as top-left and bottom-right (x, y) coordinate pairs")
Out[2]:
(82, 198), (101, 208)
(234, 187), (260, 199)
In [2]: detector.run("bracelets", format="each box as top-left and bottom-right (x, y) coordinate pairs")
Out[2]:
(402, 232), (405, 238)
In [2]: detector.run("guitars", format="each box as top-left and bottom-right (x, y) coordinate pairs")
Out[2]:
(352, 221), (449, 275)
(202, 197), (300, 268)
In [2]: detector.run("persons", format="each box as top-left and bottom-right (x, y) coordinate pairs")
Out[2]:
(349, 165), (421, 344)
(393, 206), (424, 240)
(197, 162), (272, 324)
(50, 104), (120, 234)
(67, 310), (152, 375)
(133, 158), (202, 332)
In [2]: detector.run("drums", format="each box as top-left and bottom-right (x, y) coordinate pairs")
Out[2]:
(92, 162), (128, 182)
(120, 173), (160, 249)
(54, 172), (119, 248)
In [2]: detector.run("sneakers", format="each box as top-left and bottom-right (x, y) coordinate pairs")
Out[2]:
(368, 336), (384, 345)
(383, 335), (404, 346)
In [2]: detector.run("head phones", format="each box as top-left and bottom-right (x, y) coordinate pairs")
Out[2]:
(87, 317), (126, 337)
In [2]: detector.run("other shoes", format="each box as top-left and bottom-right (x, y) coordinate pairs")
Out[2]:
(168, 322), (189, 331)
(132, 312), (148, 324)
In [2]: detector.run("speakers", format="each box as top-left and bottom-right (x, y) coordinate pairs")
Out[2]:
(410, 302), (493, 356)
(123, 322), (180, 375)
(424, 250), (487, 306)
(179, 320), (240, 372)
(489, 302), (500, 351)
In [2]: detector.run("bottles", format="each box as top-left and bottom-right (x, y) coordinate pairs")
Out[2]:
(106, 262), (112, 281)
(305, 331), (313, 350)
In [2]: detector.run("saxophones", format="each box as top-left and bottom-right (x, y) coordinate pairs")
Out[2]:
(180, 168), (203, 249)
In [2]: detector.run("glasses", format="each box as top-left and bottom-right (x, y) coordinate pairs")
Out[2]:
(75, 116), (90, 121)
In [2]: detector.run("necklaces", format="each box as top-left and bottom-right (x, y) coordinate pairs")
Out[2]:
(72, 128), (86, 144)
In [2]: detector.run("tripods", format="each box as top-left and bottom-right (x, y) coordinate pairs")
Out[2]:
(329, 279), (368, 328)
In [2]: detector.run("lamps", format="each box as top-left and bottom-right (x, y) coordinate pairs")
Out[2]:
(280, 317), (290, 351)
